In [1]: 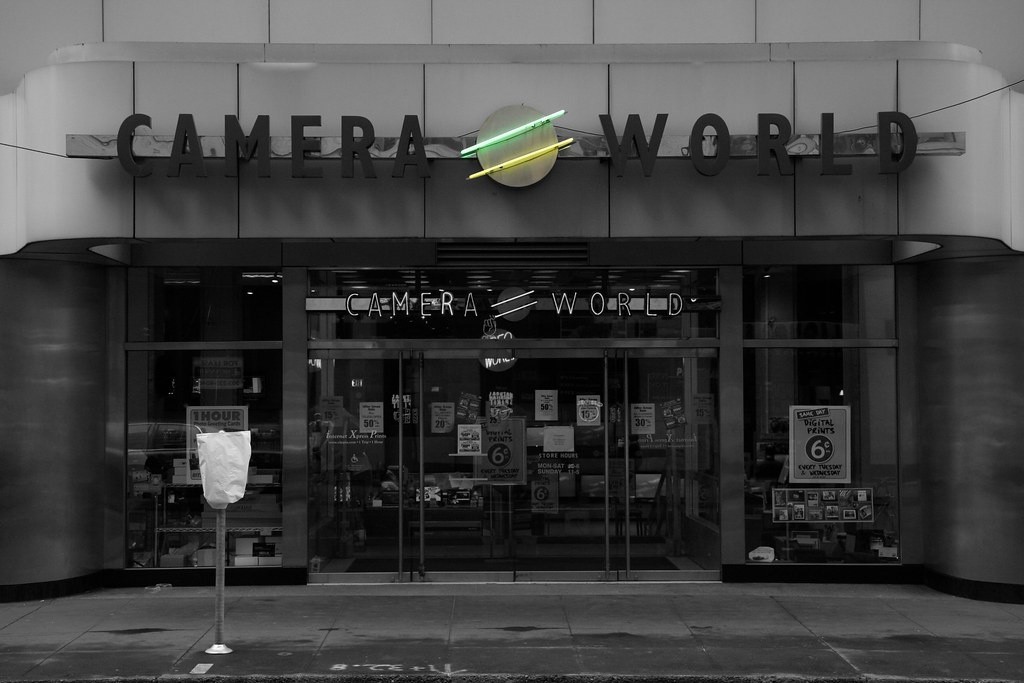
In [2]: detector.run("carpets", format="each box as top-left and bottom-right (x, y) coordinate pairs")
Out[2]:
(344, 555), (680, 572)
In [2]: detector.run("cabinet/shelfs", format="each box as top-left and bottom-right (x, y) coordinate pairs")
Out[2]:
(154, 483), (283, 568)
